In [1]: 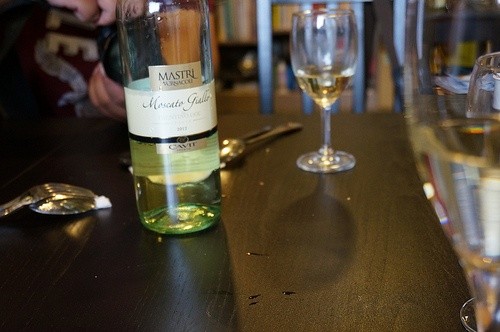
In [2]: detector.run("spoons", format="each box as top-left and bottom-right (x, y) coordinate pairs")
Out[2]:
(220, 122), (303, 163)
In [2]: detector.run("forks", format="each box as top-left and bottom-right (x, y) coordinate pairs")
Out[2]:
(0, 183), (94, 219)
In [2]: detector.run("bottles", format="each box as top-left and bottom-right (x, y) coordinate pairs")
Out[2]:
(115, 1), (223, 234)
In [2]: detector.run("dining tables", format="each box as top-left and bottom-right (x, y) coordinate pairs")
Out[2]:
(0, 105), (478, 332)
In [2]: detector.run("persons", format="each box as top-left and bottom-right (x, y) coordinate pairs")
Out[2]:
(0, 0), (145, 122)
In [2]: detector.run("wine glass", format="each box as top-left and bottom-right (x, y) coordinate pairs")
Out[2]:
(290, 8), (357, 174)
(455, 51), (500, 332)
(404, 0), (500, 332)
